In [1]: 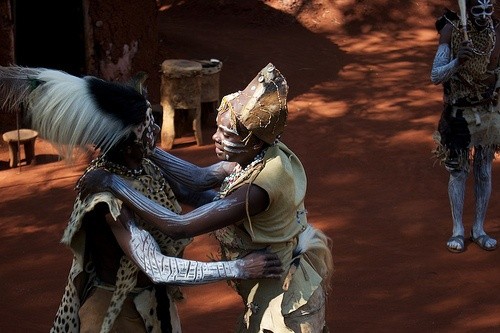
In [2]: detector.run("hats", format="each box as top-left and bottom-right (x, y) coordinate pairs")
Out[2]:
(216, 63), (289, 145)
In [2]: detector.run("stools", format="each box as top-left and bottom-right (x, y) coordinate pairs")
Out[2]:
(2, 129), (39, 167)
(160, 59), (222, 151)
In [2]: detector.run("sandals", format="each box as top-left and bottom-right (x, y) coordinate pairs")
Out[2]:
(447, 235), (465, 252)
(470, 229), (496, 250)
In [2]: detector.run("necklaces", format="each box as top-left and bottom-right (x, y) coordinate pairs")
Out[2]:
(464, 14), (497, 64)
(219, 154), (271, 191)
(99, 156), (165, 193)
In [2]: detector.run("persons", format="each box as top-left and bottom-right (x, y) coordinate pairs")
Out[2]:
(431, 0), (500, 253)
(79, 63), (333, 333)
(51, 65), (286, 333)
